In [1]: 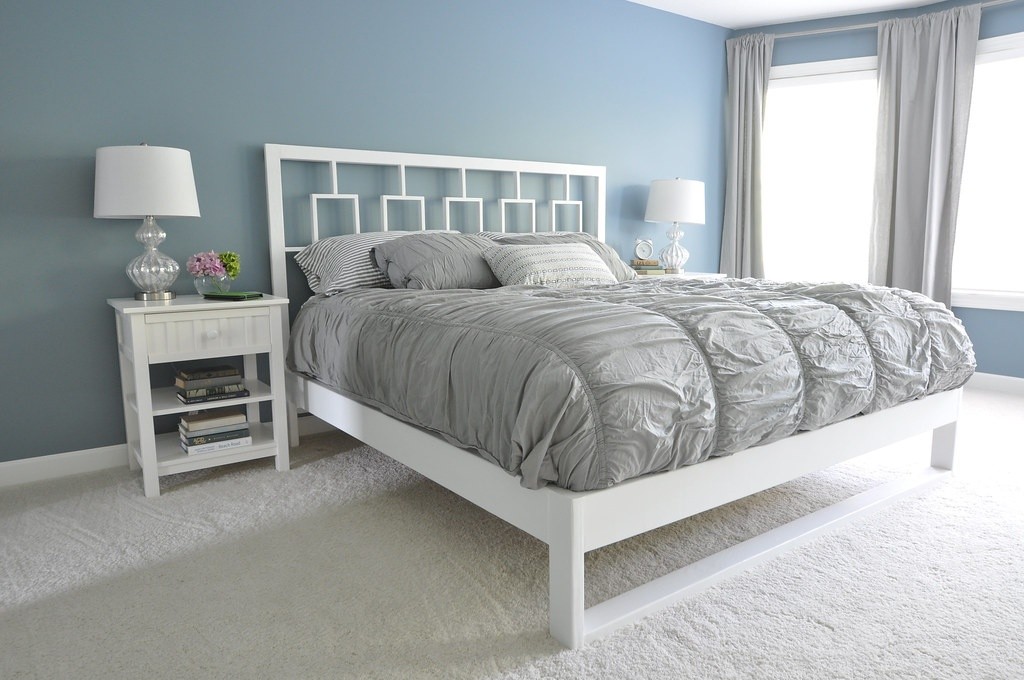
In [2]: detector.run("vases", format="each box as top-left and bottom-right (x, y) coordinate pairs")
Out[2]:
(193, 276), (230, 297)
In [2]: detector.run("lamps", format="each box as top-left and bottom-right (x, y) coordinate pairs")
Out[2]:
(643, 177), (705, 274)
(92, 142), (200, 302)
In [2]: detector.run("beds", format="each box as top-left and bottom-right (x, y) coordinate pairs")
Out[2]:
(262, 142), (977, 652)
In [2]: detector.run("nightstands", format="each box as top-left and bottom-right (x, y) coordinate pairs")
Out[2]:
(105, 292), (289, 497)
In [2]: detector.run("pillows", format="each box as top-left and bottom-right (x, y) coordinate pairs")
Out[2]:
(497, 237), (638, 282)
(371, 232), (503, 291)
(294, 230), (461, 299)
(474, 230), (592, 241)
(481, 241), (619, 293)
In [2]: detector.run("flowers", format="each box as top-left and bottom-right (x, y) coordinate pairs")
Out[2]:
(186, 249), (240, 292)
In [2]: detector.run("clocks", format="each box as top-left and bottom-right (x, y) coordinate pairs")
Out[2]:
(633, 237), (653, 260)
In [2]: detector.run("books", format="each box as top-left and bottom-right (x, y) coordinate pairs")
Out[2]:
(174, 365), (249, 405)
(630, 259), (665, 275)
(178, 409), (253, 456)
